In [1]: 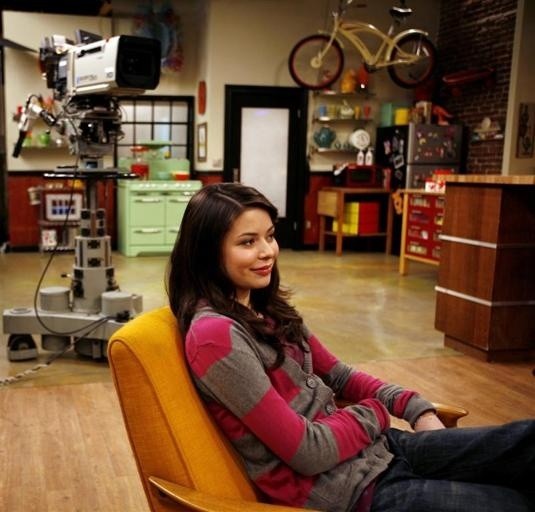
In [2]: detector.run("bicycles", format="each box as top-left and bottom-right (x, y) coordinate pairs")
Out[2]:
(288, 1), (438, 89)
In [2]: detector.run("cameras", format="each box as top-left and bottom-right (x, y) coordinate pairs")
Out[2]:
(13, 27), (161, 157)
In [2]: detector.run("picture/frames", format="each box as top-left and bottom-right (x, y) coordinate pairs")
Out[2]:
(197, 123), (207, 163)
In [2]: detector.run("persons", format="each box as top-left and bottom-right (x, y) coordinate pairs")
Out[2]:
(168, 182), (535, 512)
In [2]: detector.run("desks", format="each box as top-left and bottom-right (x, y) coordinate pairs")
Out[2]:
(432, 174), (535, 362)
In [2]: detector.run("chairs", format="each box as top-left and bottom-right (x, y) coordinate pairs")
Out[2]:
(107, 306), (469, 512)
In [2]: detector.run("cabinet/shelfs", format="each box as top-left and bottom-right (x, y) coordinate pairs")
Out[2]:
(117, 179), (204, 257)
(319, 187), (394, 255)
(7, 169), (119, 252)
(400, 189), (445, 277)
(318, 88), (377, 153)
